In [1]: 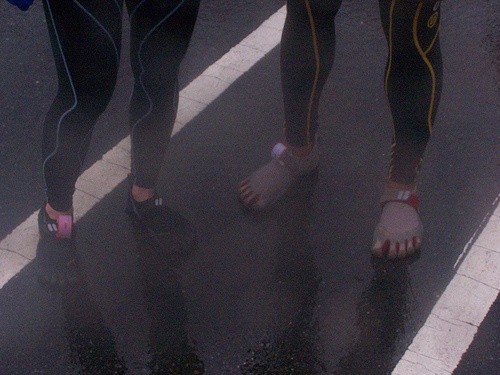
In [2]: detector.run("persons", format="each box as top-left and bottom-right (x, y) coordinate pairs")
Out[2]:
(240, 0), (444, 259)
(7, 0), (202, 288)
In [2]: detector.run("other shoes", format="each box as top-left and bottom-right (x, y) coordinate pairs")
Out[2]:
(372, 176), (425, 258)
(127, 187), (198, 257)
(239, 145), (320, 213)
(37, 206), (83, 285)
